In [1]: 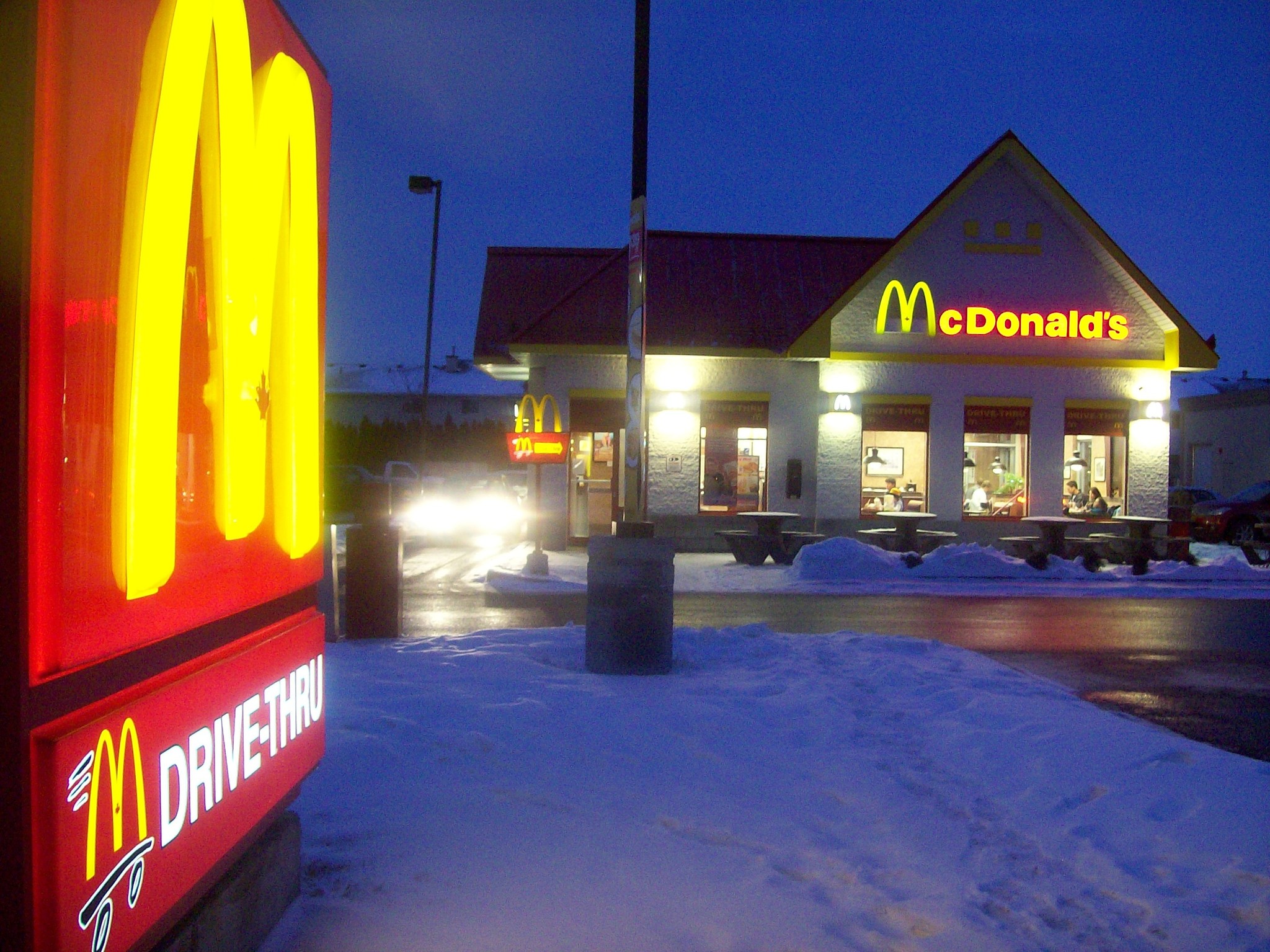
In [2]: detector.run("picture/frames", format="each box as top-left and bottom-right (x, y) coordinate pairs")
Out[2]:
(865, 447), (904, 477)
(1063, 464), (1071, 479)
(1094, 457), (1106, 482)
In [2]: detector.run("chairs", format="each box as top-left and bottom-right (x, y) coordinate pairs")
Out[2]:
(1009, 503), (1022, 516)
(906, 500), (925, 512)
(1107, 505), (1121, 517)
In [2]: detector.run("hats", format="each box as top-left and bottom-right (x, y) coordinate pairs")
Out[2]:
(889, 488), (901, 496)
(885, 477), (896, 485)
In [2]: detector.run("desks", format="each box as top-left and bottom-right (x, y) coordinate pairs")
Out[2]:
(737, 511), (800, 565)
(1112, 515), (1172, 575)
(993, 501), (1013, 514)
(1021, 516), (1087, 559)
(876, 511), (937, 552)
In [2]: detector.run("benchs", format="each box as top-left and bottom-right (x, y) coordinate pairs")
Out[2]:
(999, 536), (1102, 570)
(714, 530), (826, 564)
(1089, 532), (1193, 564)
(858, 528), (958, 551)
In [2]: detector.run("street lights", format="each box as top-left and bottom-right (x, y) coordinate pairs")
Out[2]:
(409, 176), (444, 477)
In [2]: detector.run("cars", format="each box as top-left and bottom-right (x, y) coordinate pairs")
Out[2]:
(1191, 482), (1270, 546)
(1168, 486), (1225, 510)
(354, 460), (528, 547)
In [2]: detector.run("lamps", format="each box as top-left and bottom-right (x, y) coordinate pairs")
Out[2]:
(863, 431), (886, 469)
(963, 452), (976, 472)
(1065, 435), (1087, 471)
(988, 433), (1006, 474)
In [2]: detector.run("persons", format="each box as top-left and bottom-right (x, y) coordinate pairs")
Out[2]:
(1079, 487), (1106, 511)
(969, 480), (992, 515)
(885, 477), (905, 512)
(963, 477), (984, 510)
(1084, 497), (1104, 515)
(1063, 482), (1086, 519)
